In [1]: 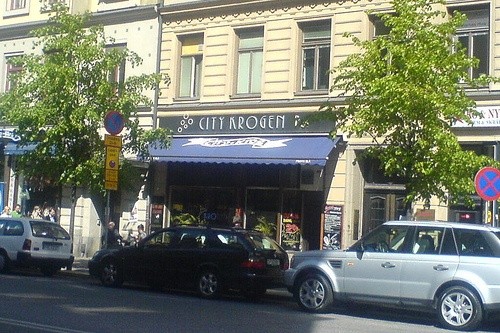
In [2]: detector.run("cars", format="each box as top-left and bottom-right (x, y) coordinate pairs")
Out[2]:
(89, 224), (289, 302)
(0, 217), (74, 277)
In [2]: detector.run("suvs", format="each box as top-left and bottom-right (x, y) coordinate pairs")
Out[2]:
(284, 220), (500, 332)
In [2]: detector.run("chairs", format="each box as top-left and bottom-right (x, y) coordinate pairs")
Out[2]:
(183, 236), (198, 249)
(417, 234), (434, 254)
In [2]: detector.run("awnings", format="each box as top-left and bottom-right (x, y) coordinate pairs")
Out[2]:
(0, 128), (150, 162)
(136, 134), (344, 167)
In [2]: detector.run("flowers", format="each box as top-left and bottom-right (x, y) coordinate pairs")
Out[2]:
(286, 224), (300, 238)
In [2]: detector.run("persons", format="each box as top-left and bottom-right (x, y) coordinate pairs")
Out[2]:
(100, 218), (181, 250)
(232, 221), (242, 229)
(0, 204), (59, 224)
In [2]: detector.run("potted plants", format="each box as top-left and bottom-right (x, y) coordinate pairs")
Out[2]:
(254, 215), (278, 236)
(170, 204), (196, 224)
